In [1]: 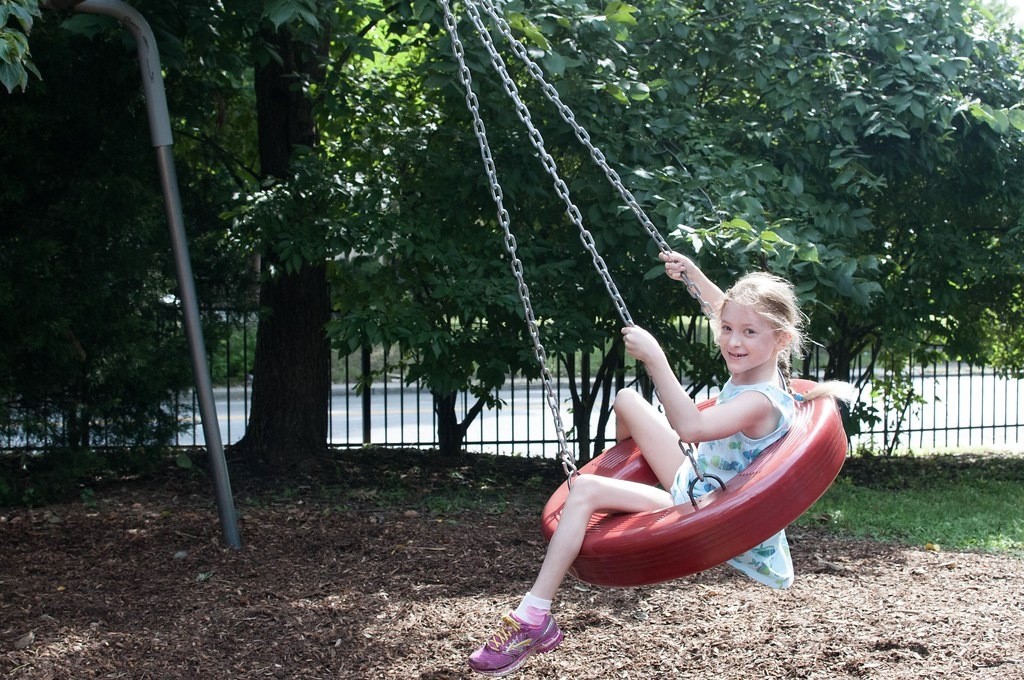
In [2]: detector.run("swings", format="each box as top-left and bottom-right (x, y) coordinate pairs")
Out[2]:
(440, 0), (847, 585)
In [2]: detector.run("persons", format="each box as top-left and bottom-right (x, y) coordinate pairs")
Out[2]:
(468, 251), (857, 676)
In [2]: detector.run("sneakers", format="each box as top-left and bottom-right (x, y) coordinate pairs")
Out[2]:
(467, 612), (563, 677)
